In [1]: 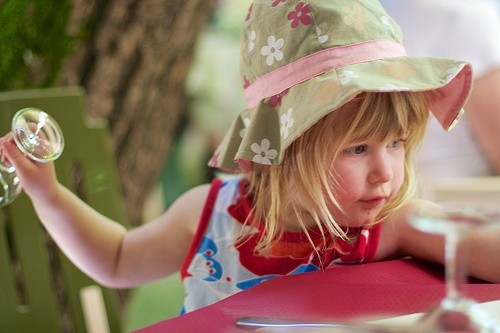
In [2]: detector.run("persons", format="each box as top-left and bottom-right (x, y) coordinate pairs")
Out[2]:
(0, 0), (500, 315)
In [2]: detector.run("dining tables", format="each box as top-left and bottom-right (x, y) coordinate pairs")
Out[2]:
(129, 255), (500, 333)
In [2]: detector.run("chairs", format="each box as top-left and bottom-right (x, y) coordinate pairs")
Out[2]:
(0, 88), (131, 333)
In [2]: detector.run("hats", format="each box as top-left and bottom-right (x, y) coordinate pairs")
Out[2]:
(207, 0), (473, 173)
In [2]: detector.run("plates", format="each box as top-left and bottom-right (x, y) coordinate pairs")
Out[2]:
(352, 300), (500, 333)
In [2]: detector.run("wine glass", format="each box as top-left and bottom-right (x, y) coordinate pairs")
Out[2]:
(402, 176), (500, 333)
(0, 107), (65, 208)
